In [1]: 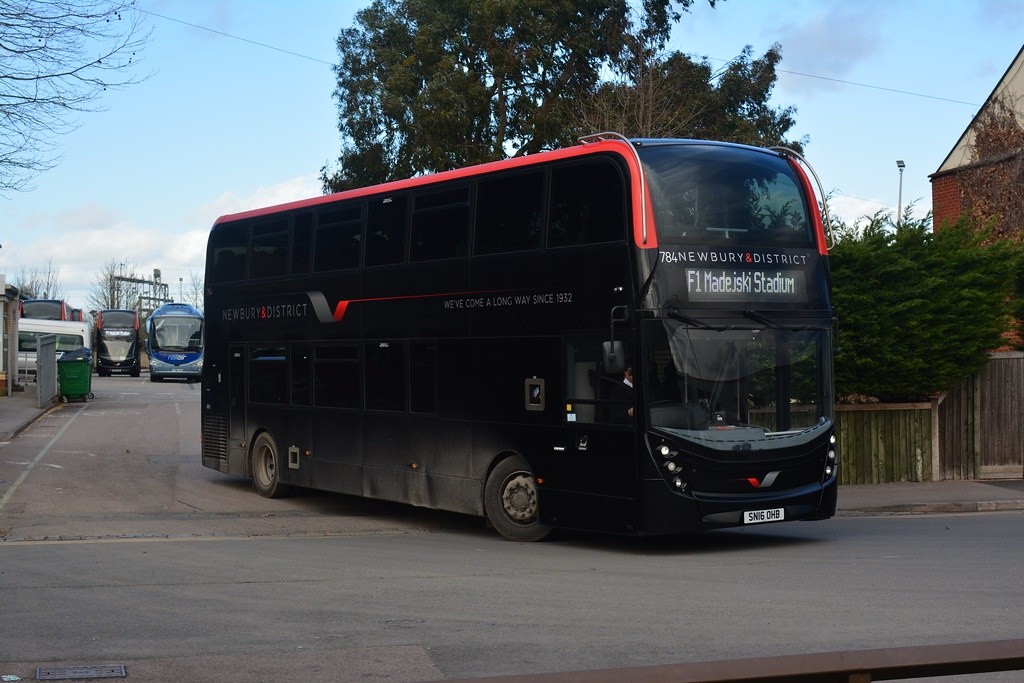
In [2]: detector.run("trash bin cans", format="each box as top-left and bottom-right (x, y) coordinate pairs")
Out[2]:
(57, 352), (95, 403)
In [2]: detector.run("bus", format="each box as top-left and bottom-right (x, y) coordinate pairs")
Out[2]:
(145, 303), (204, 382)
(201, 132), (841, 545)
(97, 310), (140, 377)
(19, 298), (93, 332)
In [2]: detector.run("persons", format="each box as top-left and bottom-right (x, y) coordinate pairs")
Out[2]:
(607, 363), (634, 424)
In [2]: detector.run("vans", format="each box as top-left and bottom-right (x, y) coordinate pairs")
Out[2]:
(17, 318), (91, 383)
